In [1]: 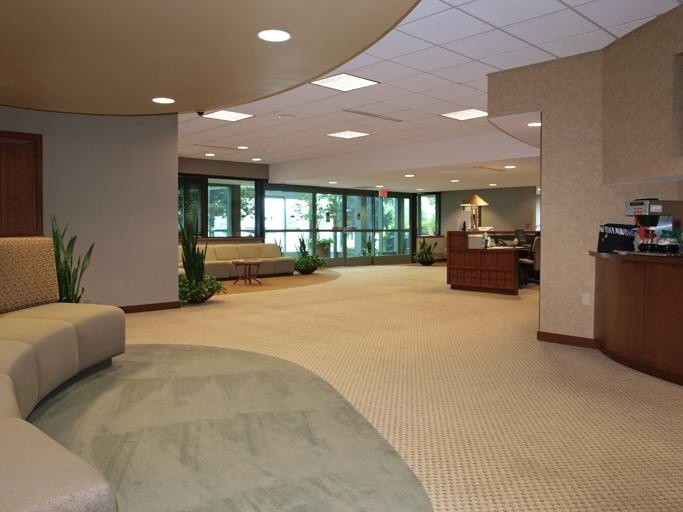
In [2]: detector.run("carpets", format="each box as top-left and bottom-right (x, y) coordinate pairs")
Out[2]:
(26, 344), (432, 511)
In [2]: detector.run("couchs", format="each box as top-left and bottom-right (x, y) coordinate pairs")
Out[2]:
(178, 243), (296, 283)
(1, 235), (128, 512)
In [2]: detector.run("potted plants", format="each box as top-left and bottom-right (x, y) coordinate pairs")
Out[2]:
(411, 237), (439, 265)
(294, 234), (333, 275)
(177, 219), (226, 303)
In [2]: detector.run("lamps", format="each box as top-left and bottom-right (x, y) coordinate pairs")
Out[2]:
(460, 194), (488, 232)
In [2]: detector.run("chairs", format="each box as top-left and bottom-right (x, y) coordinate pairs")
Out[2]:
(518, 236), (540, 288)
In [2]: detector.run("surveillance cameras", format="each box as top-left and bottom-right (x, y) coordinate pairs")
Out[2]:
(197, 112), (203, 115)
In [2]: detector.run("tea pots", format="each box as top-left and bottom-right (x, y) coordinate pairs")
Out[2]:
(637, 229), (680, 253)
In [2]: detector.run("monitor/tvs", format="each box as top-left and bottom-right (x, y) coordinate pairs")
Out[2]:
(515, 229), (527, 245)
